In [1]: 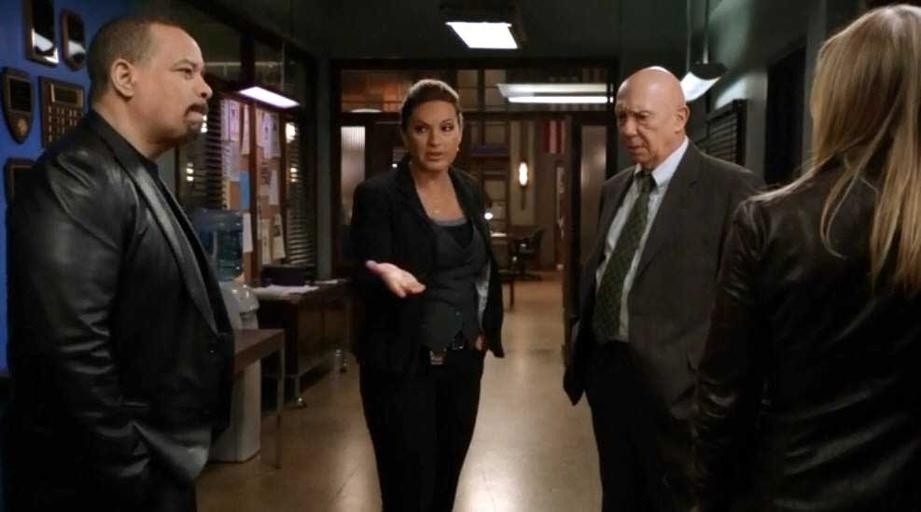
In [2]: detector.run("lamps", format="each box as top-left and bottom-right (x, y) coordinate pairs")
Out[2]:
(679, 0), (729, 103)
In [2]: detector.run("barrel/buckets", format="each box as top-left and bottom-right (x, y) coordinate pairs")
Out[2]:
(190, 207), (244, 282)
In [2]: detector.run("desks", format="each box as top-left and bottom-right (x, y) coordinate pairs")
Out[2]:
(233, 325), (287, 470)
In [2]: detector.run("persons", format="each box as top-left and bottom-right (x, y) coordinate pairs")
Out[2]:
(685, 2), (921, 512)
(0, 12), (236, 512)
(342, 77), (505, 512)
(562, 65), (770, 512)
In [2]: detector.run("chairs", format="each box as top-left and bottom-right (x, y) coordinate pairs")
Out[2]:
(507, 223), (548, 280)
(487, 233), (518, 311)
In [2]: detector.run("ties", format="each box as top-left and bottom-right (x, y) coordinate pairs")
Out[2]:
(591, 174), (656, 344)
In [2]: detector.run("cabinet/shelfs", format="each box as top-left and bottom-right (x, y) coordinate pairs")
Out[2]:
(251, 274), (356, 407)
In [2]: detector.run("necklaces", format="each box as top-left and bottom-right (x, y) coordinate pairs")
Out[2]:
(420, 175), (452, 218)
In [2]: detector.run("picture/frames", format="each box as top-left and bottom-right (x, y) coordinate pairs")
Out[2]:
(2, 155), (34, 209)
(58, 9), (88, 72)
(23, 1), (62, 67)
